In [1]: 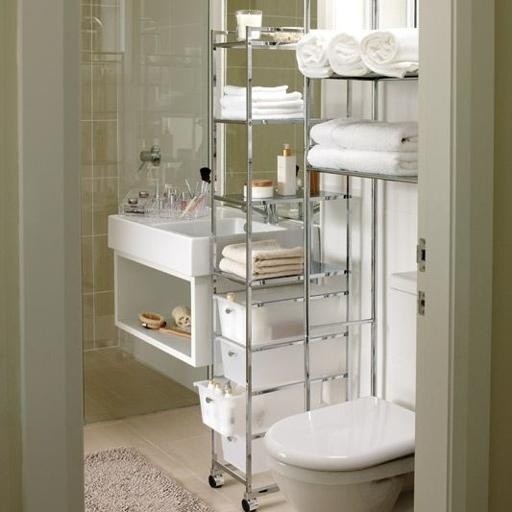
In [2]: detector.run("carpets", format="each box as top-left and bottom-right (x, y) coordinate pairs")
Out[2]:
(81, 347), (204, 423)
(81, 444), (216, 511)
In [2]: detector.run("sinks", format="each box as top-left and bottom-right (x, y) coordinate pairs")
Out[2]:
(153, 217), (289, 277)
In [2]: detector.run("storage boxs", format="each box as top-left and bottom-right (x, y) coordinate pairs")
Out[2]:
(193, 278), (347, 475)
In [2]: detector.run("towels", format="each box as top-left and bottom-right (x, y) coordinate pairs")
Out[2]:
(293, 27), (420, 80)
(217, 84), (305, 119)
(304, 116), (419, 177)
(220, 238), (305, 282)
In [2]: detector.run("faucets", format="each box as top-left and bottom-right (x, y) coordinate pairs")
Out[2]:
(242, 200), (278, 223)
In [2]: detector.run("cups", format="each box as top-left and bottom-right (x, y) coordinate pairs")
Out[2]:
(234, 10), (263, 42)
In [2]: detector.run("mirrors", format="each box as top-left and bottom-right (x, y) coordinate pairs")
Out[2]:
(219, 0), (323, 227)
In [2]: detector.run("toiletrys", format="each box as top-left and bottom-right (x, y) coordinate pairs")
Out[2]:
(243, 179), (274, 199)
(277, 144), (296, 196)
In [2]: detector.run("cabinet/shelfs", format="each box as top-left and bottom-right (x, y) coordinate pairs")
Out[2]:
(113, 248), (215, 367)
(205, 19), (351, 512)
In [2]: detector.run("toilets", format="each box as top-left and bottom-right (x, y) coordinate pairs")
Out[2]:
(263, 271), (418, 512)
